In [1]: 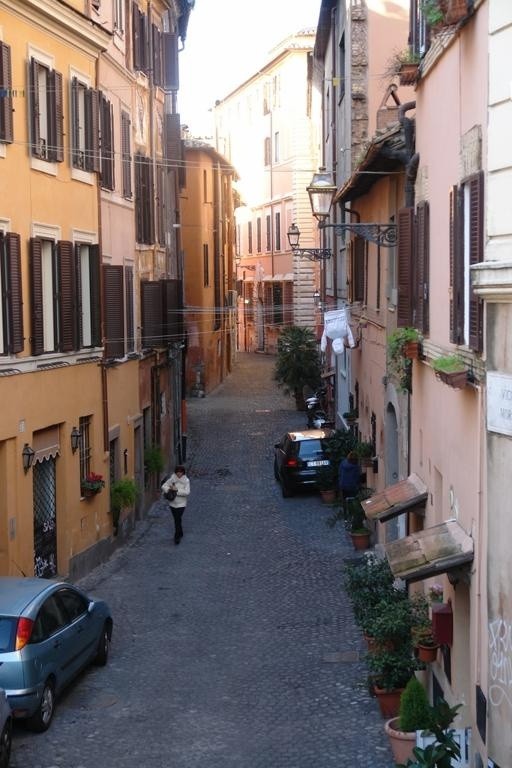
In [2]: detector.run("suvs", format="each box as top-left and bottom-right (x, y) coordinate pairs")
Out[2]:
(274, 428), (339, 497)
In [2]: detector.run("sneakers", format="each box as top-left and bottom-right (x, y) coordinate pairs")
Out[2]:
(174, 532), (183, 544)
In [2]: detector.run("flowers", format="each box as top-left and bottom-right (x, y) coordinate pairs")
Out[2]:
(83, 472), (105, 488)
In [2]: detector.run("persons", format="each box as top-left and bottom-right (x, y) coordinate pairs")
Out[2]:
(338, 449), (361, 524)
(161, 465), (190, 545)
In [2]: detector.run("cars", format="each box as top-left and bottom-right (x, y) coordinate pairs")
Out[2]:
(0, 576), (113, 732)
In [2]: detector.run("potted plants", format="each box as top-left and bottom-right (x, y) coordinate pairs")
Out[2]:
(323, 440), (460, 768)
(389, 51), (421, 85)
(386, 328), (419, 388)
(418, 0), (470, 28)
(433, 356), (466, 391)
(271, 324), (323, 410)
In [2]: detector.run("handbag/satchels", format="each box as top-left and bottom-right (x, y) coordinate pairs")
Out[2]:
(160, 474), (177, 501)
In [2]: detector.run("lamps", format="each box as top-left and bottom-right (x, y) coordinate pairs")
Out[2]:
(312, 288), (328, 312)
(286, 222), (333, 261)
(305, 166), (398, 250)
(70, 427), (82, 454)
(22, 443), (35, 475)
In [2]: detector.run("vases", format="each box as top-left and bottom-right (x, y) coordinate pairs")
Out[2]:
(87, 489), (102, 496)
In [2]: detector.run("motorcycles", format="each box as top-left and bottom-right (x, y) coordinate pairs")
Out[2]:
(302, 384), (336, 430)
